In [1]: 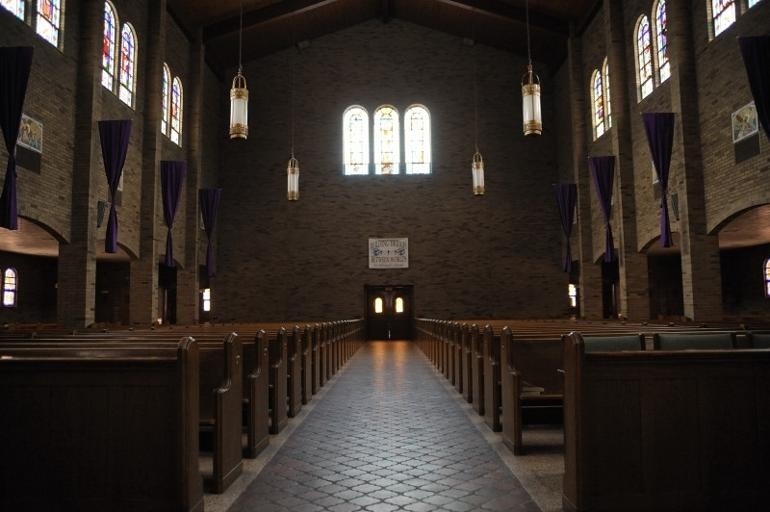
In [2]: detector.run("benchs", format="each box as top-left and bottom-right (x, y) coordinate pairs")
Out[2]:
(651, 333), (737, 350)
(500, 326), (646, 454)
(415, 317), (743, 430)
(0, 318), (365, 512)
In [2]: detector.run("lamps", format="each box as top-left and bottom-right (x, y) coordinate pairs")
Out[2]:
(521, 0), (543, 136)
(229, 0), (249, 141)
(287, 0), (299, 202)
(471, 0), (485, 196)
